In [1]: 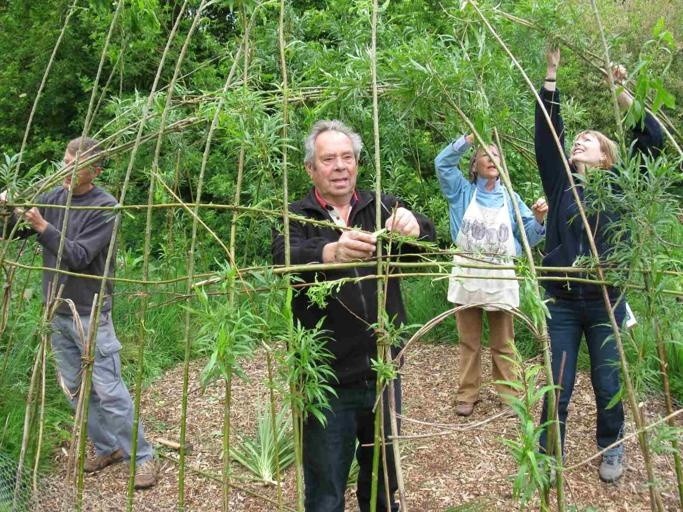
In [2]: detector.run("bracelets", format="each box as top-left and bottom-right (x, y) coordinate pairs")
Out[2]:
(544, 76), (558, 83)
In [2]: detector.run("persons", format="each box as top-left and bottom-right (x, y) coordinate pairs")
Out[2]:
(534, 31), (665, 483)
(0, 135), (161, 492)
(432, 124), (550, 417)
(269, 119), (436, 512)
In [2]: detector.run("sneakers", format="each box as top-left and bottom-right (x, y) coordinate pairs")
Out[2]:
(134, 457), (160, 490)
(84, 449), (123, 471)
(456, 401), (474, 416)
(600, 445), (623, 481)
